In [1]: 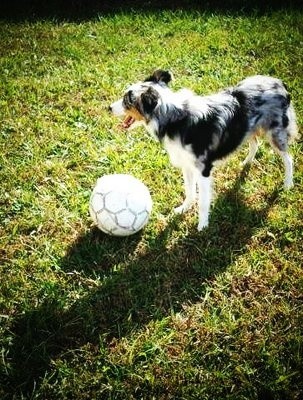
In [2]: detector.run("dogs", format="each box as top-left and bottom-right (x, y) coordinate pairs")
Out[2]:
(109, 69), (298, 232)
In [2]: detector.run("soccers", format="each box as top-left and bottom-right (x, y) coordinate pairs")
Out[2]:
(89, 174), (152, 237)
